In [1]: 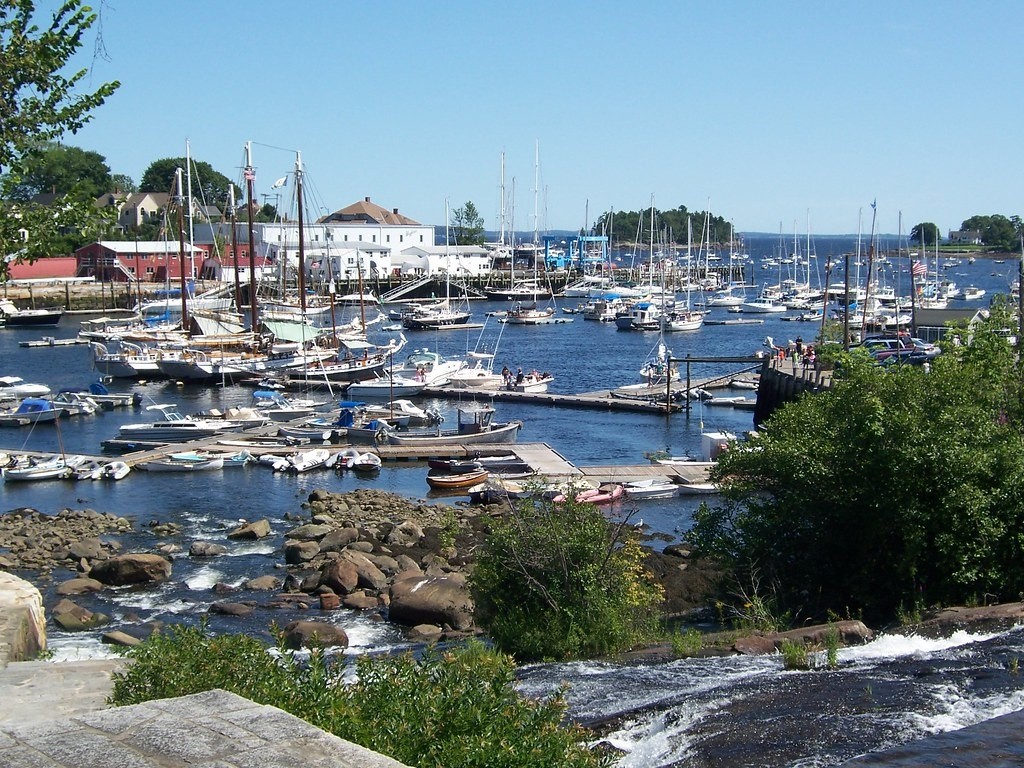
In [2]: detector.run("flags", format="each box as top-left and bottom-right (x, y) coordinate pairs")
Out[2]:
(913, 257), (927, 274)
(271, 175), (288, 189)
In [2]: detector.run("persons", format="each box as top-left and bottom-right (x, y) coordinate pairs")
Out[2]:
(655, 361), (664, 373)
(416, 367), (426, 382)
(848, 332), (860, 343)
(502, 365), (537, 390)
(779, 336), (815, 368)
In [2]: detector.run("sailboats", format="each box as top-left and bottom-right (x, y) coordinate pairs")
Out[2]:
(0, 136), (1024, 506)
